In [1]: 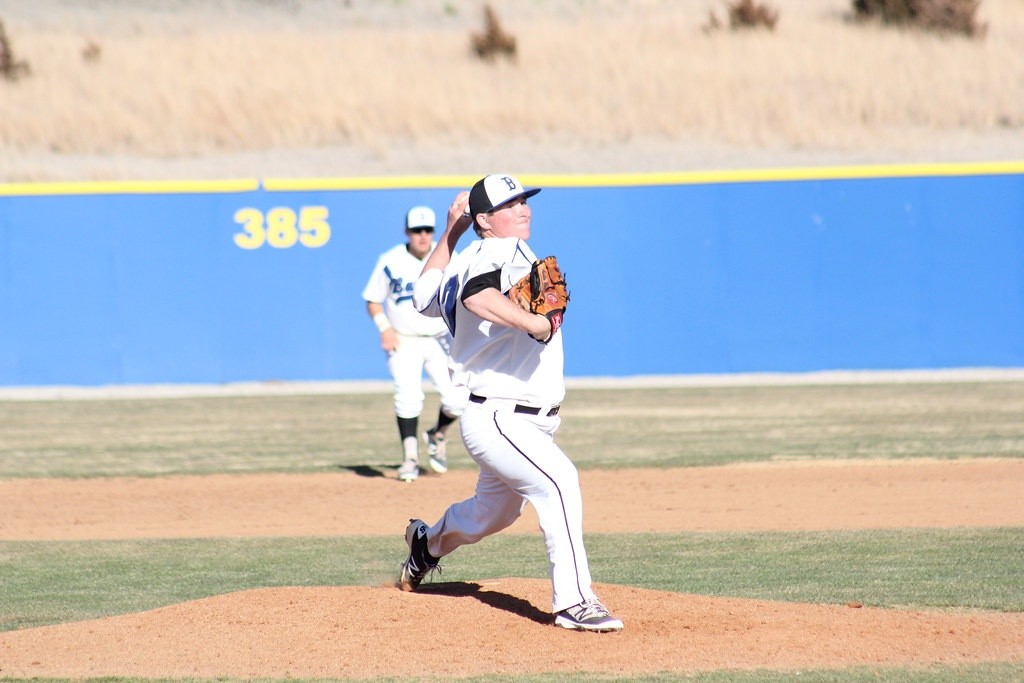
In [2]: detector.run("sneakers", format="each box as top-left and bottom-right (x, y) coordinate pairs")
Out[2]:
(399, 518), (442, 591)
(398, 458), (418, 481)
(554, 597), (623, 631)
(423, 427), (447, 474)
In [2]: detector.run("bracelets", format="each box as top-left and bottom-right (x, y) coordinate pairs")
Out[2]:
(372, 313), (390, 332)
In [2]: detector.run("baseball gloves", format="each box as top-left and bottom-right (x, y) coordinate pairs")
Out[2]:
(509, 255), (572, 347)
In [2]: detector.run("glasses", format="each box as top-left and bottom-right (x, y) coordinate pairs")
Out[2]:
(410, 226), (434, 233)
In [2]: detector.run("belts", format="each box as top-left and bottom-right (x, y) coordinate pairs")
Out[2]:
(468, 392), (560, 416)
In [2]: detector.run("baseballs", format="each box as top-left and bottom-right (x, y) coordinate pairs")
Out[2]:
(459, 190), (471, 214)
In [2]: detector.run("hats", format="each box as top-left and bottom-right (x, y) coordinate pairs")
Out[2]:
(407, 206), (436, 229)
(468, 174), (542, 219)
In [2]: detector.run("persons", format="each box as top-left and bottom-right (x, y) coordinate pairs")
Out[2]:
(362, 206), (463, 480)
(398, 175), (624, 631)
